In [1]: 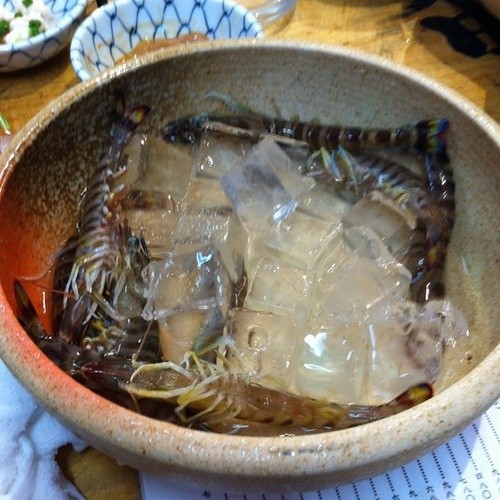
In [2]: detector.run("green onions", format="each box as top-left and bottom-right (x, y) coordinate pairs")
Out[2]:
(0, 0), (42, 43)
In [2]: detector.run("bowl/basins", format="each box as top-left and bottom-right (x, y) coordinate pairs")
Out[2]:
(70, 1), (266, 83)
(0, 36), (499, 492)
(0, 0), (86, 73)
